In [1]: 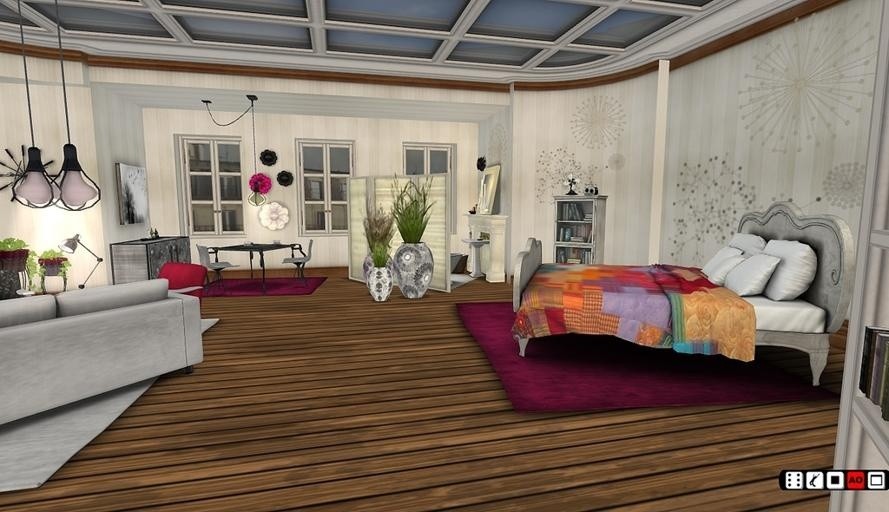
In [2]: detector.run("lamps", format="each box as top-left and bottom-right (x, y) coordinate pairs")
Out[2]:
(11, 0), (63, 210)
(49, 0), (102, 213)
(58, 233), (104, 289)
(246, 94), (266, 206)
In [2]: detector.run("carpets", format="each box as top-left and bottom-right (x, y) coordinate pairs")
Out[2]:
(0, 317), (223, 493)
(450, 274), (479, 291)
(186, 276), (328, 297)
(453, 300), (841, 415)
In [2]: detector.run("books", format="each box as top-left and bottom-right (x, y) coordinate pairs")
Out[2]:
(555, 200), (593, 266)
(856, 325), (888, 423)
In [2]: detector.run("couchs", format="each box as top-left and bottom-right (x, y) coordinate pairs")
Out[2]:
(0, 277), (206, 427)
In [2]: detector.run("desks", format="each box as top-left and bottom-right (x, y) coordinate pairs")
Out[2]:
(462, 239), (489, 279)
(215, 244), (293, 291)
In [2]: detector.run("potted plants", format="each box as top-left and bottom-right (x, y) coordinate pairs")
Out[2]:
(38, 250), (71, 286)
(0, 236), (39, 291)
(360, 169), (438, 303)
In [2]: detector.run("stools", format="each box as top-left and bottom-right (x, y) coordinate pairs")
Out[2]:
(451, 253), (468, 274)
(40, 274), (68, 295)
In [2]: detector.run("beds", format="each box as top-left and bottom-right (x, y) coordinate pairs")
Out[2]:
(509, 199), (857, 389)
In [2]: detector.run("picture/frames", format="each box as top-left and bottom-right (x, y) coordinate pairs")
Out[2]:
(476, 165), (501, 215)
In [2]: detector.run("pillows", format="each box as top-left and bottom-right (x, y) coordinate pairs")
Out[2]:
(708, 254), (748, 285)
(727, 231), (767, 257)
(761, 238), (818, 301)
(722, 253), (782, 297)
(698, 245), (744, 279)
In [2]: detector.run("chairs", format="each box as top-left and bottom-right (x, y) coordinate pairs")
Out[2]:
(195, 243), (239, 289)
(158, 262), (207, 308)
(283, 239), (313, 287)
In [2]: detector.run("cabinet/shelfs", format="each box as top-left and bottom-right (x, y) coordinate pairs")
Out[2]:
(109, 235), (191, 285)
(553, 194), (607, 264)
(827, 1), (888, 512)
